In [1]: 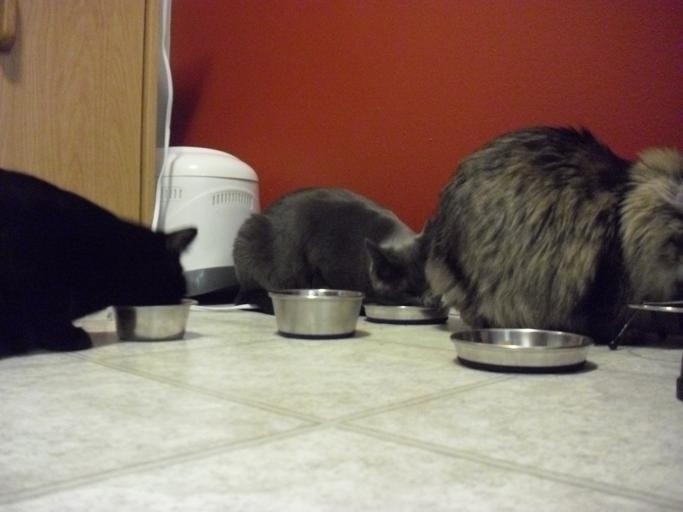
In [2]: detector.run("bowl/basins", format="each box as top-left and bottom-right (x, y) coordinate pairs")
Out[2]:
(449, 327), (595, 373)
(362, 302), (450, 324)
(113, 297), (199, 343)
(265, 287), (367, 339)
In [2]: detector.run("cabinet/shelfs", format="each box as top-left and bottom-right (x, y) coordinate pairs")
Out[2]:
(2, 0), (164, 231)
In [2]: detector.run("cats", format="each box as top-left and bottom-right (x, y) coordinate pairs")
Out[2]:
(231, 183), (433, 312)
(414, 120), (683, 345)
(0, 167), (197, 358)
